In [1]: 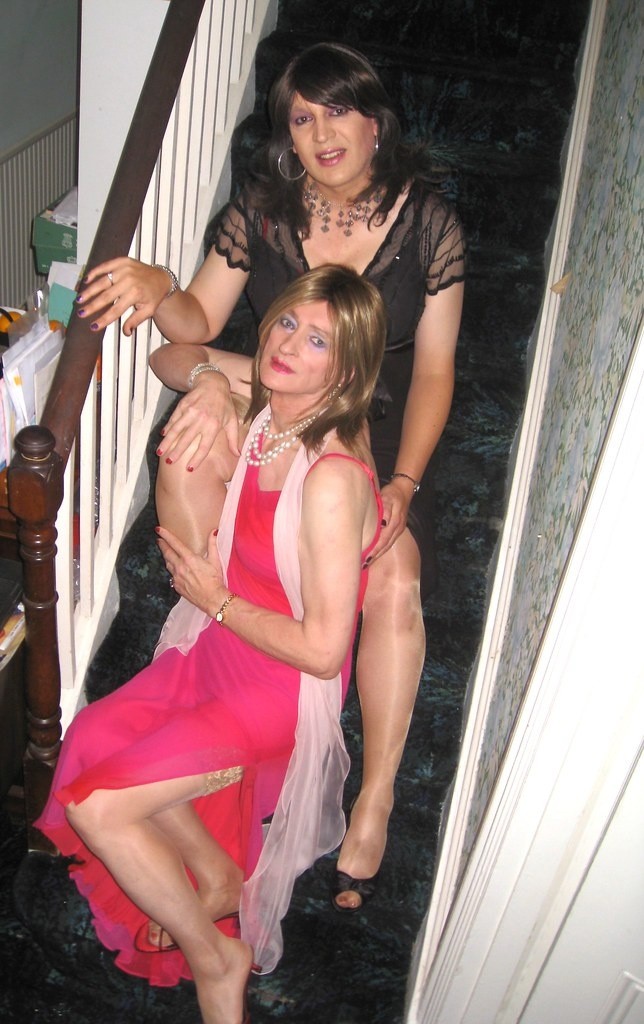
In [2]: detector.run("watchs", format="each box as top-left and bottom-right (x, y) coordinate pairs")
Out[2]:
(215, 593), (240, 626)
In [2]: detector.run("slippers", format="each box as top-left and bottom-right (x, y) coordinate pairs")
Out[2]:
(242, 975), (251, 1023)
(133, 905), (248, 952)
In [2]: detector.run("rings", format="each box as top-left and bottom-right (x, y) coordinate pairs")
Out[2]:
(169, 577), (174, 587)
(107, 272), (114, 286)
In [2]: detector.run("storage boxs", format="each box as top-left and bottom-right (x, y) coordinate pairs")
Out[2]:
(31, 184), (78, 274)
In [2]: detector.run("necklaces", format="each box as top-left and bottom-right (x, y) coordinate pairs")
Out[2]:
(304, 178), (383, 236)
(244, 413), (319, 466)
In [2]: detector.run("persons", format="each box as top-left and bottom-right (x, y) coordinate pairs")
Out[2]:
(75, 40), (471, 914)
(32, 263), (383, 1024)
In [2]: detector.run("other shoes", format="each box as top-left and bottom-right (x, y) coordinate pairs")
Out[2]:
(327, 793), (398, 916)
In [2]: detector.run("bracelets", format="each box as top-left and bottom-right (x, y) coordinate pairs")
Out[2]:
(188, 362), (223, 391)
(149, 263), (178, 298)
(390, 473), (421, 493)
